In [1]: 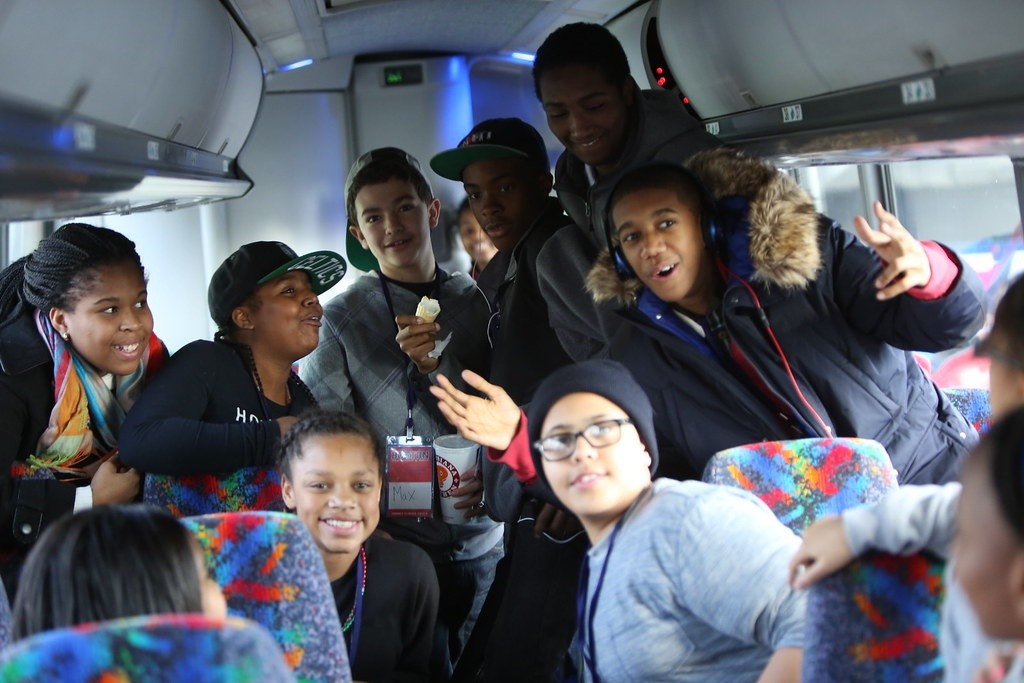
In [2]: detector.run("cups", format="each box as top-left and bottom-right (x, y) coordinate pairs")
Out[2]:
(433, 434), (481, 525)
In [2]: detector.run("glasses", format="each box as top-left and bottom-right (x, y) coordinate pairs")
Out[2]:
(532, 418), (634, 461)
(971, 338), (1024, 369)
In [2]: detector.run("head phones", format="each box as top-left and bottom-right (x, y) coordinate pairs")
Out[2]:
(603, 160), (726, 282)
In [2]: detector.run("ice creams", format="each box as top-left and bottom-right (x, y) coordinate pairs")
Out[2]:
(416, 296), (441, 323)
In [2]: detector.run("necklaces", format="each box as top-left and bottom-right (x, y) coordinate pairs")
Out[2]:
(577, 507), (628, 682)
(238, 343), (320, 421)
(341, 546), (366, 634)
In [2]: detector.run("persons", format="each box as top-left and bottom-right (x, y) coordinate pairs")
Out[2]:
(268, 409), (440, 683)
(527, 357), (811, 683)
(10, 503), (228, 642)
(532, 23), (729, 364)
(300, 147), (504, 674)
(458, 195), (500, 285)
(0, 222), (172, 613)
(429, 145), (988, 516)
(118, 242), (346, 484)
(788, 270), (1024, 682)
(429, 117), (588, 682)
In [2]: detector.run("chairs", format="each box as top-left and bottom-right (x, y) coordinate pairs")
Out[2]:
(0, 510), (353, 683)
(698, 438), (943, 682)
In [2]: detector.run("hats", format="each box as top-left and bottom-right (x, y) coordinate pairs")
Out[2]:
(346, 147), (434, 272)
(209, 241), (347, 326)
(527, 359), (660, 515)
(430, 117), (550, 177)
